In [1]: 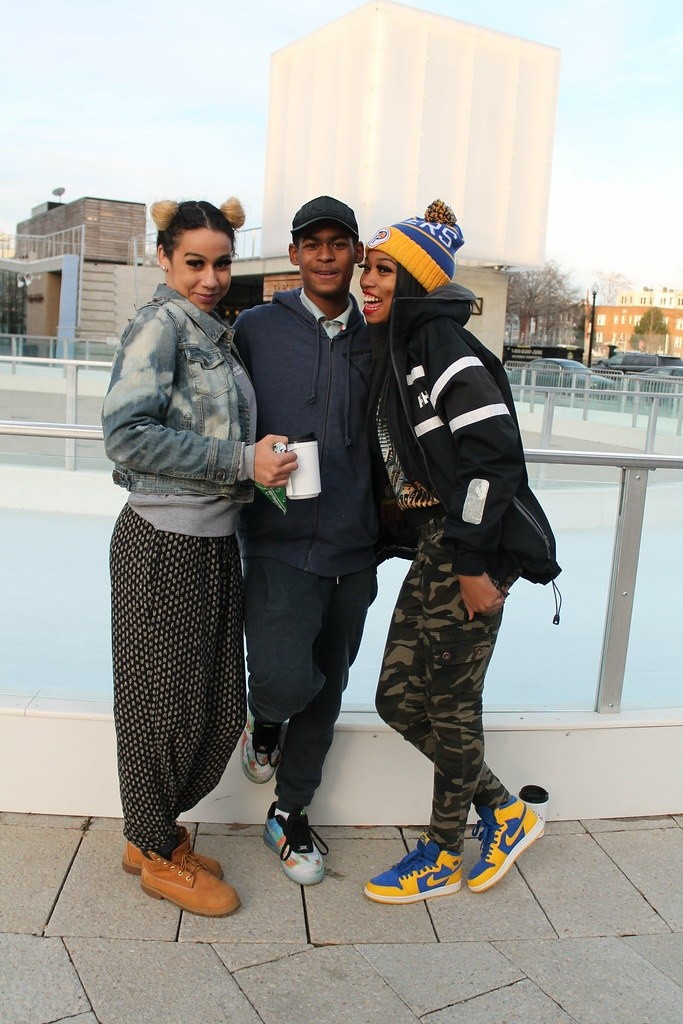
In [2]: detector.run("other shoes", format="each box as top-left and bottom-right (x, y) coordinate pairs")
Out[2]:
(122, 826), (224, 880)
(140, 833), (241, 918)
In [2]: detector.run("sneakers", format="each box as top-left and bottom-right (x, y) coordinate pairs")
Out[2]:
(263, 801), (329, 885)
(241, 701), (282, 784)
(363, 830), (463, 905)
(467, 795), (545, 892)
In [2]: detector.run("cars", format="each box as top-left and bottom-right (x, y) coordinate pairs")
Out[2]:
(623, 365), (683, 385)
(511, 358), (617, 401)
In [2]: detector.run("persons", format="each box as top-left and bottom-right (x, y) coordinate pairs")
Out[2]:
(224, 195), (402, 888)
(357, 198), (563, 904)
(98, 195), (300, 918)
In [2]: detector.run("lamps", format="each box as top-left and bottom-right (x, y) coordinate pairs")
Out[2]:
(16, 274), (24, 288)
(24, 272), (41, 286)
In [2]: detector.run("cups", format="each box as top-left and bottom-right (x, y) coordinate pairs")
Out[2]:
(282, 437), (322, 499)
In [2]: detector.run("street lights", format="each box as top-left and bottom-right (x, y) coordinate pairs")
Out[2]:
(588, 281), (599, 368)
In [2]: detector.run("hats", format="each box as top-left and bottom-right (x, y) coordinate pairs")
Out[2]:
(366, 199), (465, 293)
(290, 196), (359, 238)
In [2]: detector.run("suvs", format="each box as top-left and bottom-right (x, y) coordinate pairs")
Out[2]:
(592, 349), (683, 372)
